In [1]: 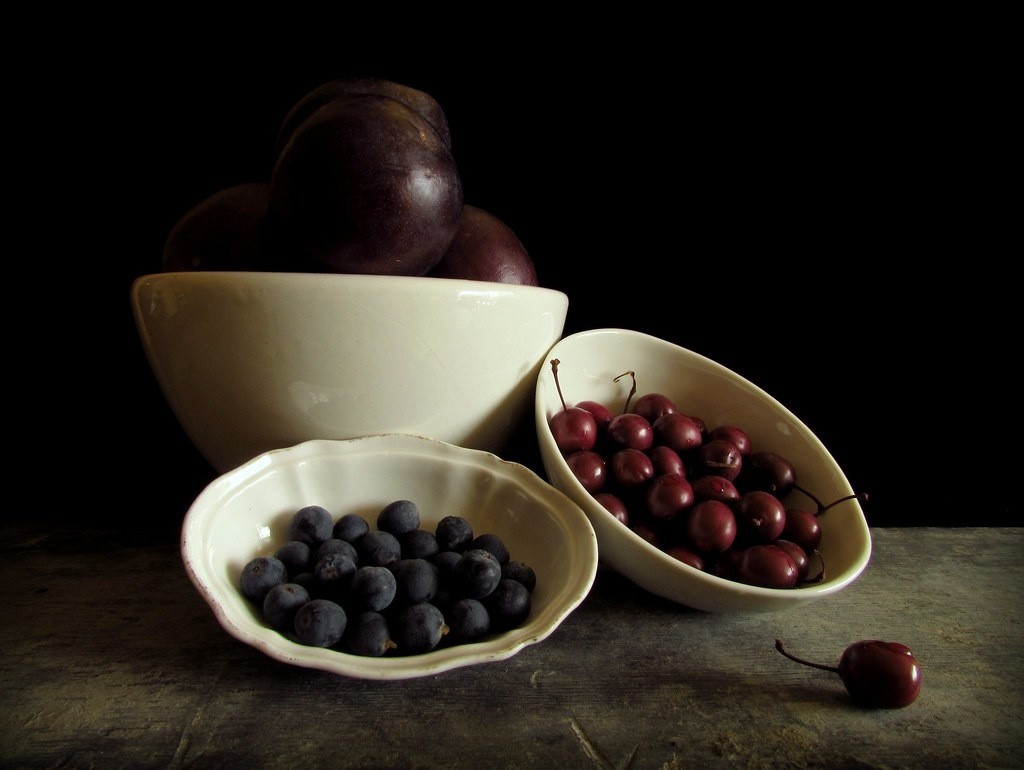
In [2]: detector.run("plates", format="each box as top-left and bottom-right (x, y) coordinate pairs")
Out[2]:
(180, 433), (600, 682)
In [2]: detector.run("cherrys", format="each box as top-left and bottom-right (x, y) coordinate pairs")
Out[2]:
(551, 357), (869, 588)
(775, 640), (921, 708)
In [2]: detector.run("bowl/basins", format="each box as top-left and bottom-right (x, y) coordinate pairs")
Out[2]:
(536, 326), (873, 615)
(131, 273), (570, 476)
